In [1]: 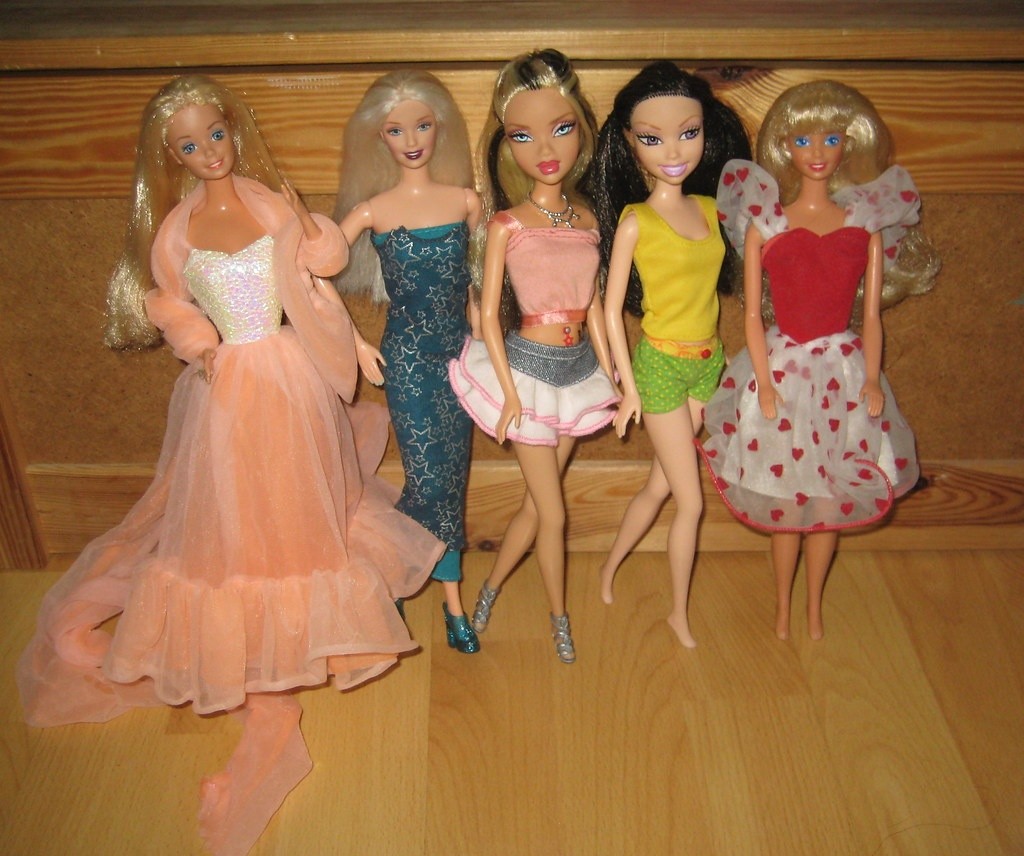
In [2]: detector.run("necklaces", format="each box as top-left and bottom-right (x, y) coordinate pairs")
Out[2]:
(526, 190), (580, 229)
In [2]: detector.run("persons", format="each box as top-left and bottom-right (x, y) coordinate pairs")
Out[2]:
(310, 68), (488, 653)
(693, 80), (940, 639)
(450, 50), (626, 664)
(577, 61), (752, 648)
(14, 71), (450, 856)
